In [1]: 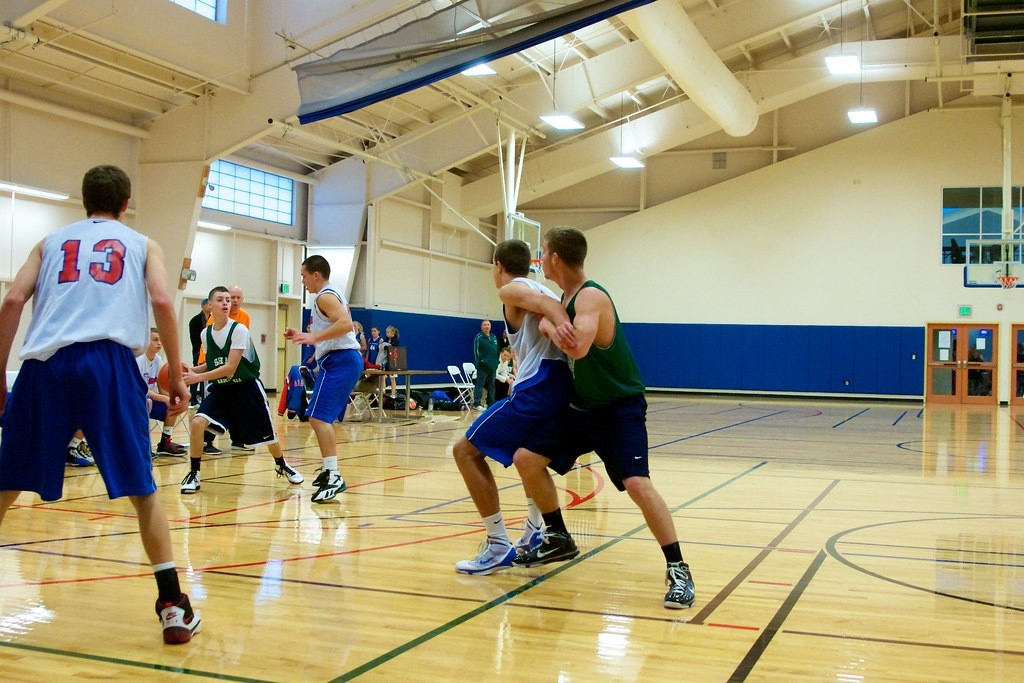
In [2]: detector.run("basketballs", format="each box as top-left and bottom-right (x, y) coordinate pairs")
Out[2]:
(157, 362), (188, 393)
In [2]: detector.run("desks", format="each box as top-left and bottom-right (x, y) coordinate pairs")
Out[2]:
(360, 369), (447, 425)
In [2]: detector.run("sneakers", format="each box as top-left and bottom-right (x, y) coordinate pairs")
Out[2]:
(275, 462), (305, 485)
(65, 443), (98, 466)
(180, 469), (202, 493)
(511, 530), (580, 568)
(455, 535), (517, 576)
(513, 520), (548, 556)
(311, 467), (348, 501)
(157, 442), (188, 456)
(663, 561), (696, 609)
(230, 441), (255, 451)
(155, 592), (203, 644)
(151, 451), (157, 459)
(203, 442), (223, 454)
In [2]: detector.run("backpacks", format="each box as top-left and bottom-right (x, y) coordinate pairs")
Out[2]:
(383, 390), (467, 411)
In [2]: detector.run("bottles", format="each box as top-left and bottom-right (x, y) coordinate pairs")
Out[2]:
(428, 399), (433, 412)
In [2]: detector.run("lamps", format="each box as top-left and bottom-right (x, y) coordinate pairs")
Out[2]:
(824, 0), (862, 76)
(847, 70), (879, 124)
(610, 94), (647, 169)
(461, 25), (498, 77)
(202, 177), (215, 191)
(540, 38), (586, 131)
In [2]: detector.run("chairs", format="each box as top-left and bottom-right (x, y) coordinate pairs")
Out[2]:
(447, 365), (476, 411)
(297, 365), (386, 420)
(462, 362), (476, 406)
(150, 405), (200, 439)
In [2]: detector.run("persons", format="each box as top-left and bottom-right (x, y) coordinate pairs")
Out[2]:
(495, 328), (518, 402)
(198, 286), (254, 454)
(304, 322), (319, 379)
(366, 327), (384, 365)
(381, 325), (399, 396)
(0, 165), (200, 645)
(179, 286), (304, 493)
(353, 321), (367, 353)
(951, 339), (985, 395)
(135, 328), (187, 459)
(453, 238), (575, 576)
(189, 299), (211, 406)
(1017, 343), (1024, 397)
(284, 255), (364, 500)
(473, 320), (500, 411)
(65, 428), (95, 466)
(513, 227), (697, 608)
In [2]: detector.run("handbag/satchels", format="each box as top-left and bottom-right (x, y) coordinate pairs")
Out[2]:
(345, 395), (371, 422)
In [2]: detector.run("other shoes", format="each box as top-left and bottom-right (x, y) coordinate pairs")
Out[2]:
(191, 404), (201, 409)
(473, 405), (486, 411)
